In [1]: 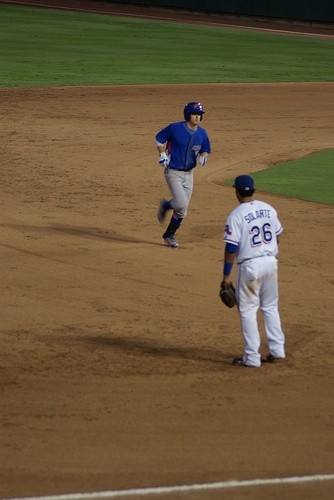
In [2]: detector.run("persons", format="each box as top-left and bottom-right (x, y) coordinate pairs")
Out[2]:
(219, 175), (285, 368)
(155, 102), (211, 246)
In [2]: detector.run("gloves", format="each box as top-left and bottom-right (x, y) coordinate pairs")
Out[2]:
(199, 152), (209, 166)
(159, 152), (171, 167)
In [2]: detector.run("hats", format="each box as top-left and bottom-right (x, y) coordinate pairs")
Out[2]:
(232, 174), (256, 190)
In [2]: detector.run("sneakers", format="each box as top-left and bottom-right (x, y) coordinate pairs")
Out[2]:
(232, 358), (244, 366)
(158, 199), (167, 222)
(163, 234), (179, 248)
(267, 353), (280, 361)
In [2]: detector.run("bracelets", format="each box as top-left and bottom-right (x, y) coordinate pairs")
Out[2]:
(223, 262), (232, 275)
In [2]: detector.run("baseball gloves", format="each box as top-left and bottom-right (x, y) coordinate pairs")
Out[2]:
(219, 280), (237, 308)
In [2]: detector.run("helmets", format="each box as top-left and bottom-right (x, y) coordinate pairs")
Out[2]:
(184, 102), (205, 122)
